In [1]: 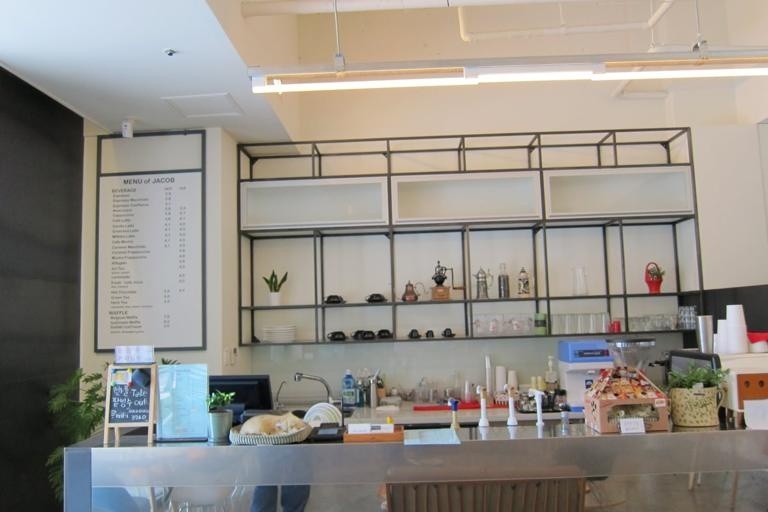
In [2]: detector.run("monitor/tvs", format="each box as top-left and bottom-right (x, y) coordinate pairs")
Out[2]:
(208, 374), (273, 411)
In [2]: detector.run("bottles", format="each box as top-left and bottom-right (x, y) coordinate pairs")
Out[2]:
(356, 367), (386, 406)
(559, 412), (569, 425)
(498, 262), (510, 298)
(545, 356), (558, 384)
(517, 267), (530, 297)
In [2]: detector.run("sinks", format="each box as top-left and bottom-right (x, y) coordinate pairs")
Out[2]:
(276, 408), (353, 420)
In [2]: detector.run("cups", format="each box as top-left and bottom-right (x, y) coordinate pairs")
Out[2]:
(420, 355), (530, 403)
(551, 304), (749, 355)
(572, 264), (588, 296)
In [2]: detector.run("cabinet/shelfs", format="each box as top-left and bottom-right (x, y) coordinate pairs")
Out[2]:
(236, 125), (707, 348)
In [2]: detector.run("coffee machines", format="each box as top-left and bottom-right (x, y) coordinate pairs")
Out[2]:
(556, 339), (614, 411)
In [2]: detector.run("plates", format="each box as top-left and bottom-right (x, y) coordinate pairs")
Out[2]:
(263, 325), (296, 343)
(304, 402), (342, 428)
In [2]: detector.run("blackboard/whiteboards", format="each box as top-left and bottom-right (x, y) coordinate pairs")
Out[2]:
(104, 364), (156, 428)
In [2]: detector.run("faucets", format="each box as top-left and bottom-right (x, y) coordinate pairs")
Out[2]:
(294, 372), (333, 407)
(272, 381), (289, 409)
